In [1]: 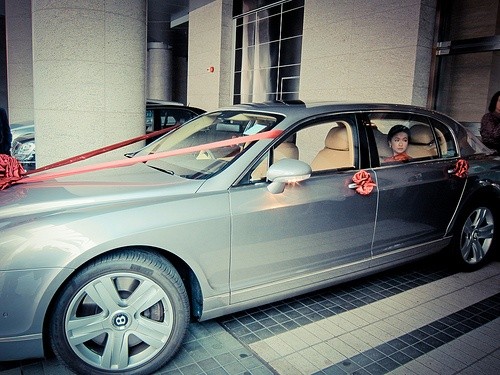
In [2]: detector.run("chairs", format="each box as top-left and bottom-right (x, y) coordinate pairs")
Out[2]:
(250, 134), (300, 181)
(310, 128), (354, 171)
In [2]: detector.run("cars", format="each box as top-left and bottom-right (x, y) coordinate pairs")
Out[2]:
(8, 99), (206, 169)
(0, 97), (500, 375)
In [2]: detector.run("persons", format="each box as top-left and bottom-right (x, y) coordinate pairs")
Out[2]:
(480, 90), (500, 152)
(382, 125), (413, 163)
(0, 107), (12, 157)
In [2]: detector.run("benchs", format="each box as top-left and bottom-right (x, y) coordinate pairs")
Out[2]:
(376, 125), (440, 163)
